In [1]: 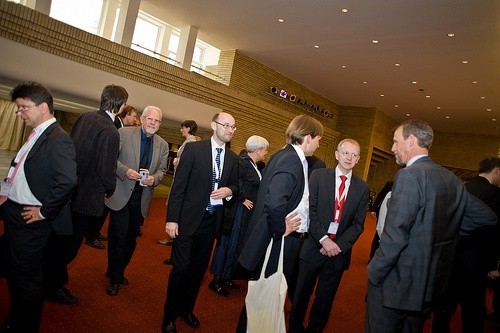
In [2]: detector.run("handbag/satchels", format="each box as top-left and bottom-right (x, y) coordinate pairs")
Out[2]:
(243, 238), (289, 332)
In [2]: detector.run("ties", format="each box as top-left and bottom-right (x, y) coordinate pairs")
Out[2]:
(329, 176), (348, 240)
(0, 130), (36, 206)
(207, 148), (223, 214)
(140, 138), (151, 168)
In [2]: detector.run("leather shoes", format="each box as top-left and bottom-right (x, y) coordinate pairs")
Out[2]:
(46, 287), (78, 305)
(162, 319), (175, 333)
(87, 238), (105, 249)
(180, 311), (200, 327)
(108, 278), (119, 295)
(224, 280), (240, 289)
(119, 276), (129, 285)
(99, 231), (109, 241)
(208, 280), (229, 295)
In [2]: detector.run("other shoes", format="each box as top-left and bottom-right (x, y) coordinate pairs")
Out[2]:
(156, 236), (174, 244)
(165, 259), (173, 263)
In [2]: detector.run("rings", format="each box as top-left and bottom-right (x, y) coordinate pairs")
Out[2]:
(31, 215), (33, 218)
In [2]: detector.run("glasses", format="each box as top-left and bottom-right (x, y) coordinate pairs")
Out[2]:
(143, 116), (162, 125)
(213, 120), (237, 132)
(15, 101), (49, 113)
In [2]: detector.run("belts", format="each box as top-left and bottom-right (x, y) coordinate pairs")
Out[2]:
(290, 230), (310, 240)
(133, 188), (143, 193)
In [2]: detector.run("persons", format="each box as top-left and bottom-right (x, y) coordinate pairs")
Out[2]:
(431, 157), (500, 333)
(364, 180), (394, 302)
(365, 122), (498, 333)
(236, 114), (324, 333)
(208, 135), (269, 297)
(288, 139), (369, 333)
(284, 154), (326, 304)
(162, 112), (239, 333)
(44, 85), (128, 304)
(104, 106), (170, 296)
(0, 82), (79, 333)
(157, 119), (201, 246)
(84, 106), (138, 249)
